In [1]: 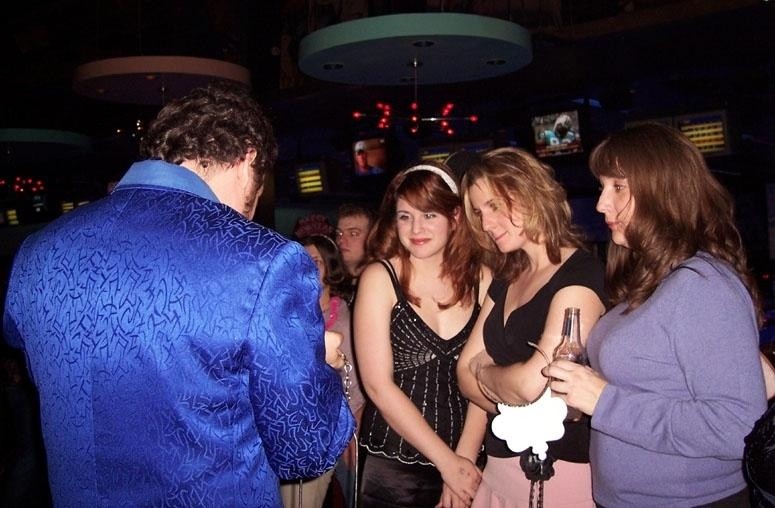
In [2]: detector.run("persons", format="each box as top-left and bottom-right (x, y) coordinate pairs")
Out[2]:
(355, 141), (383, 174)
(456, 148), (612, 508)
(3, 86), (356, 508)
(353, 159), (496, 508)
(543, 125), (775, 508)
(281, 203), (377, 508)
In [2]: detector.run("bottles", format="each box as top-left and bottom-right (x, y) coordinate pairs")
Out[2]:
(551, 308), (589, 423)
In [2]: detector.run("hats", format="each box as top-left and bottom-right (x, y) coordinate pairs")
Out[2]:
(354, 141), (368, 152)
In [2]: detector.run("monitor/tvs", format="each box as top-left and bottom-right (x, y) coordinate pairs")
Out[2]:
(349, 127), (397, 178)
(414, 141), (452, 165)
(454, 139), (497, 158)
(292, 159), (328, 198)
(0, 188), (92, 226)
(526, 107), (587, 161)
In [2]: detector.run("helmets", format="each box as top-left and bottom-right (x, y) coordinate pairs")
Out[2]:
(553, 114), (572, 130)
